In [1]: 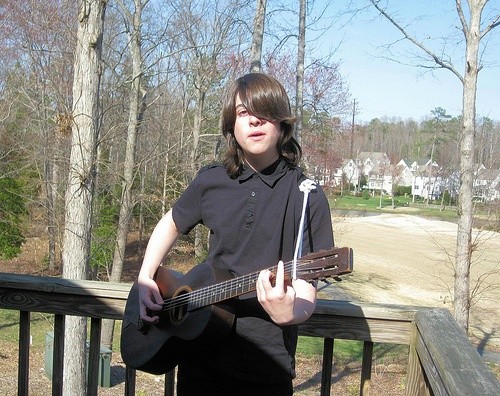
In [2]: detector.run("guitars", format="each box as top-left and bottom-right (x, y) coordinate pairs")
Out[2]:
(120, 246), (353, 376)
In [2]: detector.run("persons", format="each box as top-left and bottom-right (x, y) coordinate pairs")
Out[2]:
(136, 72), (335, 396)
